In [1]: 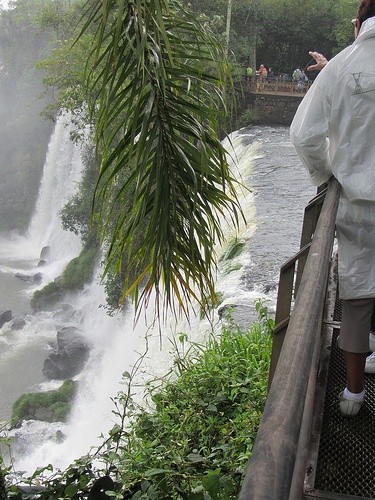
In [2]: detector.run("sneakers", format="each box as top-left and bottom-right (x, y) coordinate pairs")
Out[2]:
(338, 386), (365, 416)
(364, 351), (375, 373)
(369, 331), (375, 350)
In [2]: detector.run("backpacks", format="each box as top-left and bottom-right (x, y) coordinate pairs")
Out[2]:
(295, 69), (301, 81)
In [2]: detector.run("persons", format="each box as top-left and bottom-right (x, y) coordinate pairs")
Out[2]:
(292, 66), (308, 91)
(245, 66), (253, 87)
(256, 63), (275, 91)
(290, 0), (375, 416)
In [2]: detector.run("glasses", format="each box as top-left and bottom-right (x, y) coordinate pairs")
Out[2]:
(352, 19), (357, 25)
